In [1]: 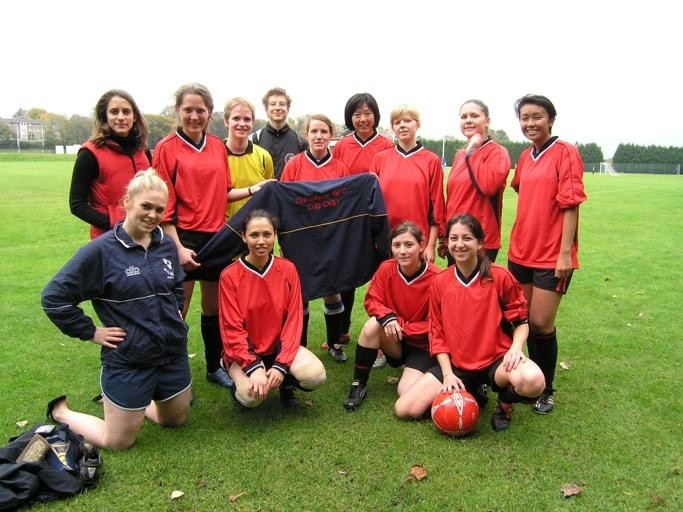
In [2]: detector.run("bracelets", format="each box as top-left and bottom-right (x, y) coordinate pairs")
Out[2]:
(248, 187), (253, 197)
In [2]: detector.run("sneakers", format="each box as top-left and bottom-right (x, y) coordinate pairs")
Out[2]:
(207, 367), (233, 388)
(343, 379), (368, 409)
(320, 334), (350, 349)
(532, 385), (554, 414)
(492, 399), (514, 432)
(280, 384), (297, 403)
(329, 343), (347, 361)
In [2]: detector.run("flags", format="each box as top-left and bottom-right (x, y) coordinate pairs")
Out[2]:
(183, 169), (393, 302)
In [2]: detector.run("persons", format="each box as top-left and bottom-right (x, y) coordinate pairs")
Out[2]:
(214, 95), (276, 214)
(39, 167), (195, 452)
(149, 84), (280, 388)
(333, 93), (396, 349)
(280, 114), (346, 361)
(435, 100), (512, 265)
(365, 104), (444, 367)
(65, 86), (156, 246)
(507, 93), (585, 416)
(218, 209), (324, 412)
(346, 220), (447, 406)
(395, 213), (544, 431)
(240, 87), (305, 188)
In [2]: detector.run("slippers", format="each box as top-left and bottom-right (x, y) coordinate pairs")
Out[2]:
(46, 394), (66, 422)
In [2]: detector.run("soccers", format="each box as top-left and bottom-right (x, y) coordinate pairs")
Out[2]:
(431, 389), (480, 435)
